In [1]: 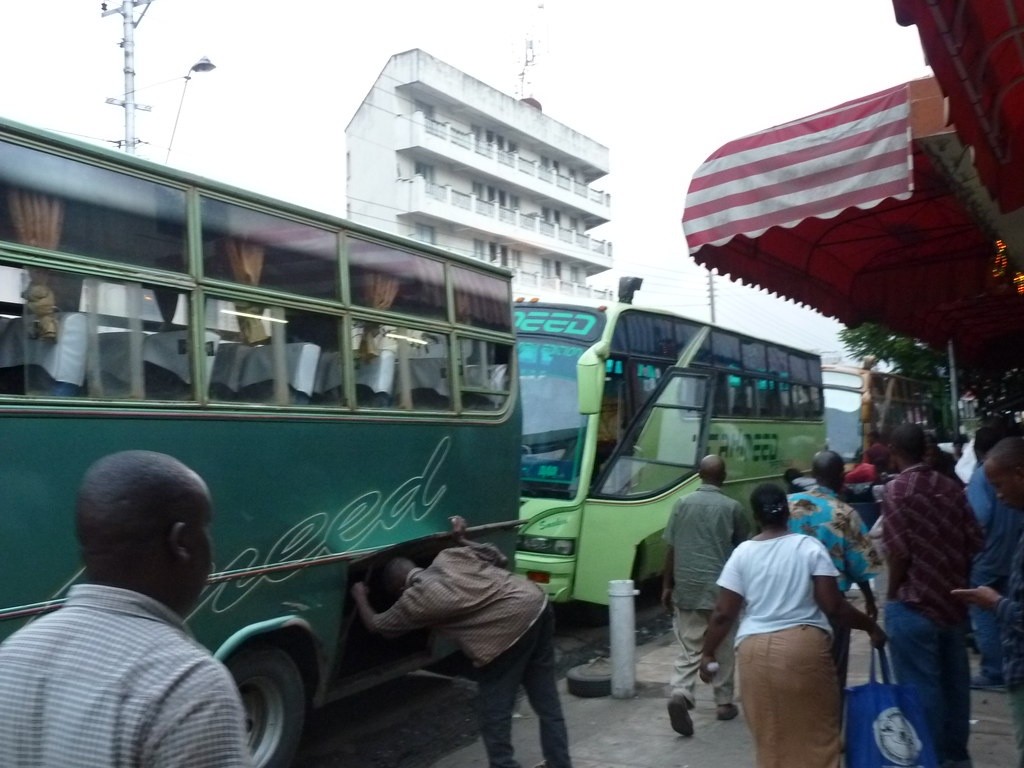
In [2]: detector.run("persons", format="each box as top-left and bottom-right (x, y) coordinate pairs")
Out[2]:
(658, 453), (754, 738)
(780, 403), (1024, 609)
(1, 448), (260, 768)
(948, 431), (1024, 768)
(348, 514), (575, 768)
(783, 449), (879, 754)
(697, 481), (893, 768)
(886, 419), (986, 768)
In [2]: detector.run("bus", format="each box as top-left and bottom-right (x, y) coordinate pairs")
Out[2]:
(821, 367), (953, 524)
(1, 115), (524, 768)
(511, 277), (829, 612)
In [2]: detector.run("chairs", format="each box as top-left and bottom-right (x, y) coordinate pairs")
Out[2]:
(3, 311), (506, 419)
(641, 373), (815, 419)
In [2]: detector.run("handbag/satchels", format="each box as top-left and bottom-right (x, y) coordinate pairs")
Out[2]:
(846, 639), (949, 768)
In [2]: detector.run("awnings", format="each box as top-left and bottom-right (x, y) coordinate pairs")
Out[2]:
(678, 80), (1024, 385)
(888, 0), (1023, 216)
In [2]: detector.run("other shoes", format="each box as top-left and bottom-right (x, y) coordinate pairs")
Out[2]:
(669, 695), (693, 736)
(716, 704), (739, 720)
